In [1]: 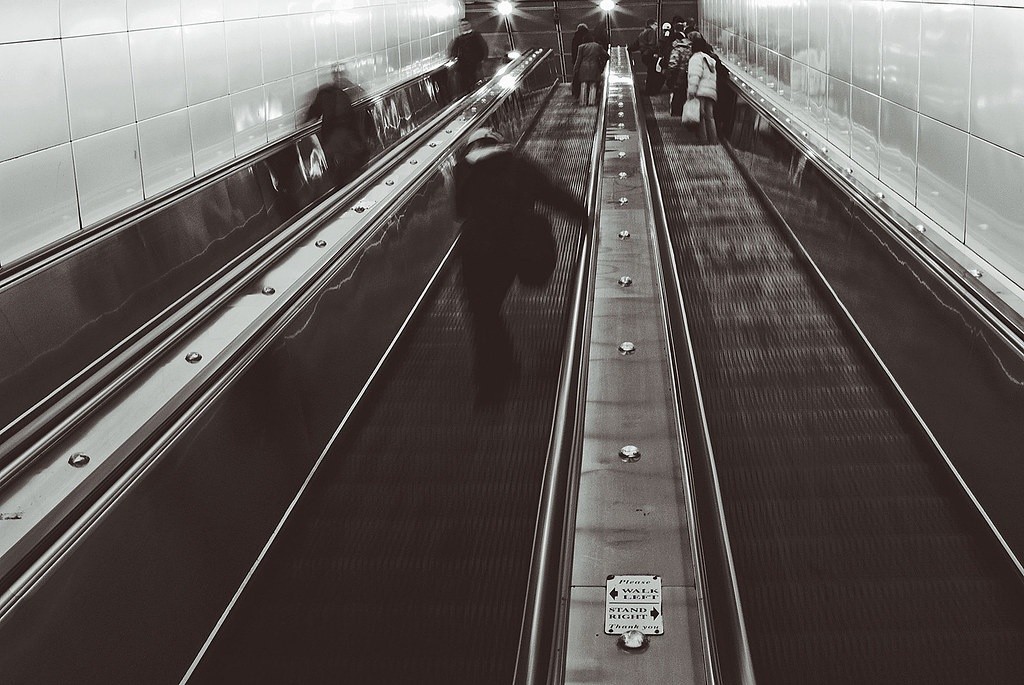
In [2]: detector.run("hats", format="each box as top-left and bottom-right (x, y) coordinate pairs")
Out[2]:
(466, 127), (504, 144)
(662, 22), (671, 29)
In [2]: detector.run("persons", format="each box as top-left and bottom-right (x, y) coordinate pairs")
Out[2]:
(447, 17), (489, 102)
(627, 15), (732, 145)
(571, 20), (610, 108)
(450, 127), (589, 407)
(297, 63), (377, 189)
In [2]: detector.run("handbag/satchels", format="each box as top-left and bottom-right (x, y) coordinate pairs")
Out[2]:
(681, 96), (700, 123)
